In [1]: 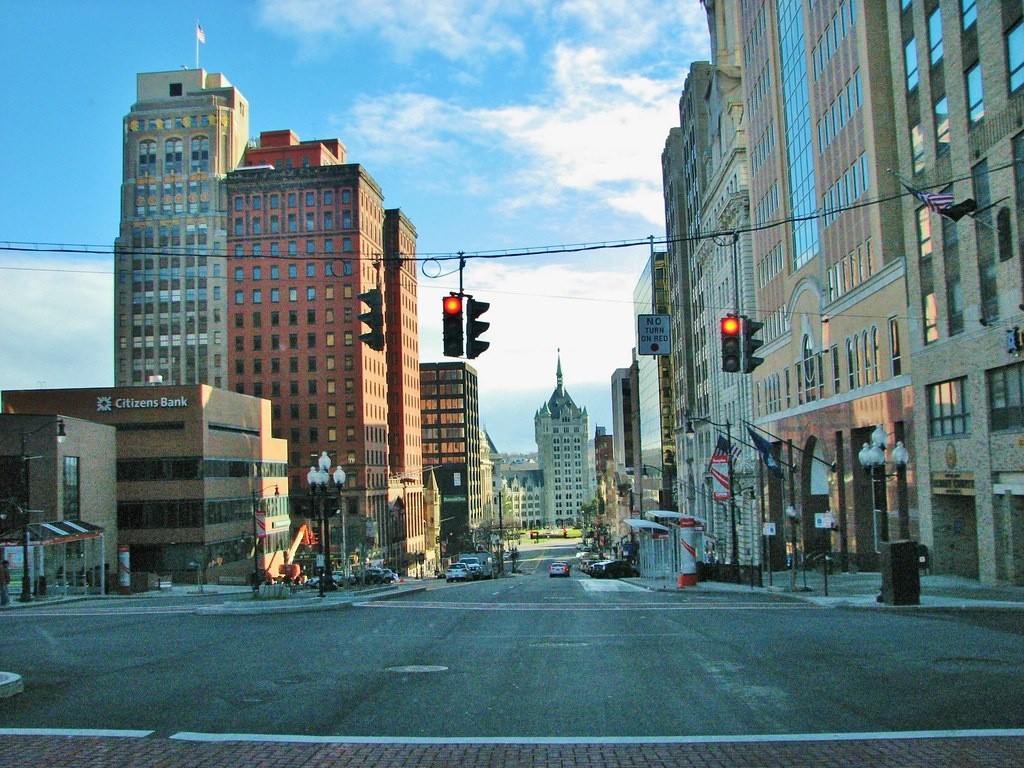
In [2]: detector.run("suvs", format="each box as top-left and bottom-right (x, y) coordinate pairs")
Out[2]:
(459, 558), (484, 580)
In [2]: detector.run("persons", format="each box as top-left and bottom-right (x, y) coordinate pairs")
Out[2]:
(0, 560), (11, 605)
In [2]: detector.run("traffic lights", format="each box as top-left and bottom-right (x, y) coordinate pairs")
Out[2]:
(357, 289), (383, 351)
(466, 298), (490, 359)
(721, 317), (743, 373)
(443, 297), (463, 357)
(743, 318), (764, 374)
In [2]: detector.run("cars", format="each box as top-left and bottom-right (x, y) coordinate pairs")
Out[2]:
(446, 563), (474, 583)
(550, 561), (571, 578)
(307, 568), (398, 589)
(576, 542), (634, 578)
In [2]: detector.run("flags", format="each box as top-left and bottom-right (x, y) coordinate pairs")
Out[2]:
(746, 427), (778, 469)
(707, 435), (742, 473)
(897, 179), (954, 214)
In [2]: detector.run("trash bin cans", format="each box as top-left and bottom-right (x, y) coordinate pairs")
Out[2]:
(33, 576), (46, 595)
(878, 541), (920, 605)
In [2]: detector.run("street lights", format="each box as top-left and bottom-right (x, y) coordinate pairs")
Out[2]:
(251, 485), (279, 580)
(20, 419), (67, 601)
(686, 417), (741, 583)
(641, 463), (673, 512)
(496, 477), (504, 529)
(859, 424), (910, 546)
(306, 452), (346, 591)
